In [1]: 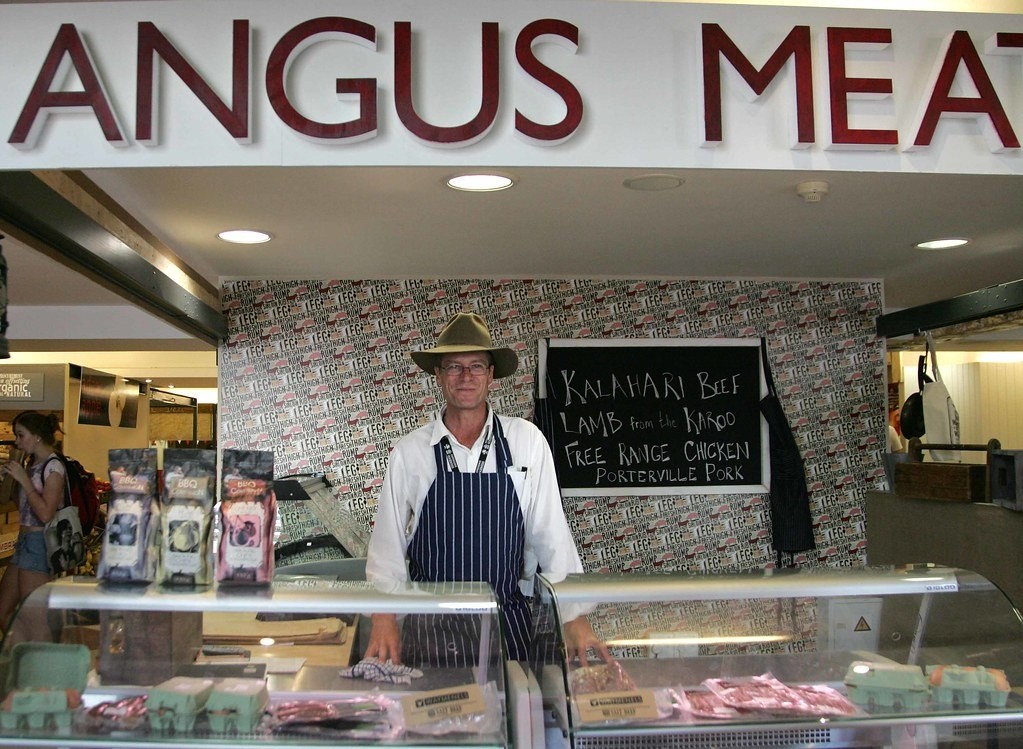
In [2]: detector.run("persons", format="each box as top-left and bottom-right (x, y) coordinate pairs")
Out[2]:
(0, 408), (106, 586)
(888, 404), (903, 453)
(0, 413), (66, 637)
(364, 312), (614, 669)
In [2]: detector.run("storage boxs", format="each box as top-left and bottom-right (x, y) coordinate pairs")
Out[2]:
(0, 641), (91, 730)
(843, 661), (929, 710)
(204, 677), (271, 733)
(142, 676), (215, 732)
(924, 664), (1011, 707)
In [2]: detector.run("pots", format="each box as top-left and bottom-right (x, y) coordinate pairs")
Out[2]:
(900, 355), (926, 440)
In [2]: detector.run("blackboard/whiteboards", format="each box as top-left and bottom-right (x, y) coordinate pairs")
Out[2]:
(532, 335), (771, 497)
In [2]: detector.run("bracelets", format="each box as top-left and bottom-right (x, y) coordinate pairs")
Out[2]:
(26, 488), (36, 498)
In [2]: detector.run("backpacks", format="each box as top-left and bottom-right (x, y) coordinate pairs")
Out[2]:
(41, 449), (100, 537)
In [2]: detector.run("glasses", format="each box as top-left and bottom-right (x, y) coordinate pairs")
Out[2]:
(440, 362), (492, 376)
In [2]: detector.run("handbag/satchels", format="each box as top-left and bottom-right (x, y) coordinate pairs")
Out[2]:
(43, 505), (87, 576)
(921, 333), (961, 462)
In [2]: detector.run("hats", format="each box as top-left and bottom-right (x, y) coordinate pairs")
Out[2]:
(409, 312), (518, 379)
